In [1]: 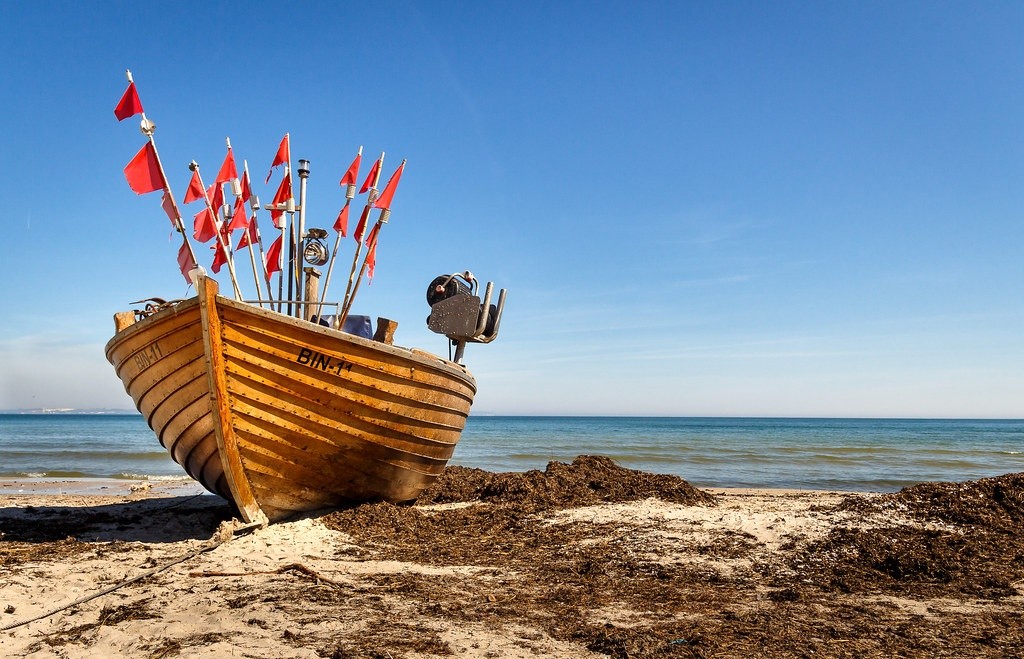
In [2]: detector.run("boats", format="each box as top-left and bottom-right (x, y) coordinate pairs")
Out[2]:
(105, 66), (507, 535)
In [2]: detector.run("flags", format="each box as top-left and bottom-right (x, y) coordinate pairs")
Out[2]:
(114, 80), (402, 283)
